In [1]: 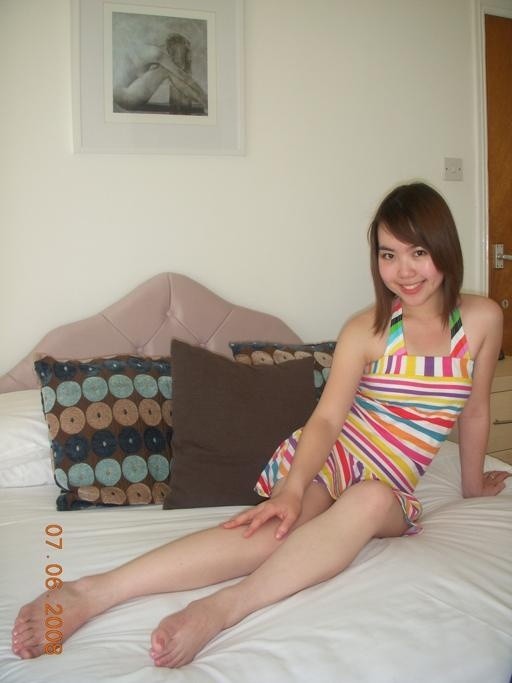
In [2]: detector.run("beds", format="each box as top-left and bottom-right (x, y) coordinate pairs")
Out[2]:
(0, 269), (510, 681)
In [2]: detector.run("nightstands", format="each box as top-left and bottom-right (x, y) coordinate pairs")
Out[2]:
(448, 354), (511, 464)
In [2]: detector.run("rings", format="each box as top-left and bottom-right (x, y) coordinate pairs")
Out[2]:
(490, 473), (497, 479)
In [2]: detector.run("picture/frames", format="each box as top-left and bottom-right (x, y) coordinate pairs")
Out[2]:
(67, 0), (247, 158)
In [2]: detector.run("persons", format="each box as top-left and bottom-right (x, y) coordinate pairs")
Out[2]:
(114, 33), (208, 114)
(11, 182), (512, 669)
